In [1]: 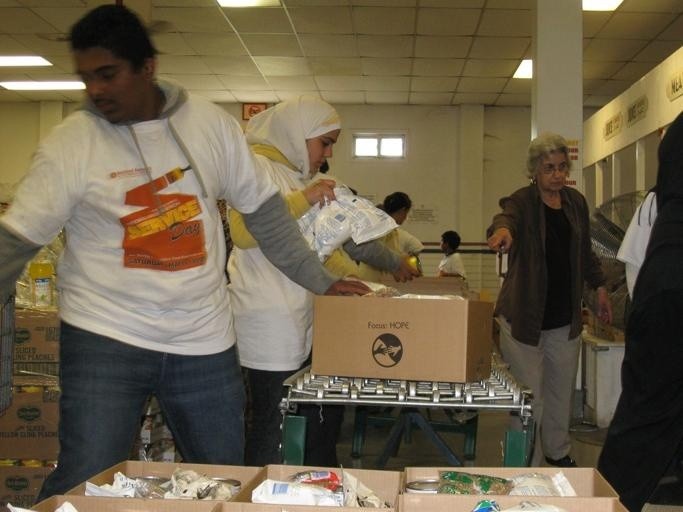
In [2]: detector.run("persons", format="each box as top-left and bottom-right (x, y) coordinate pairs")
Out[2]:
(486, 133), (613, 468)
(598, 110), (683, 512)
(0, 5), (373, 505)
(617, 185), (658, 301)
(225, 96), (346, 467)
(319, 161), (467, 284)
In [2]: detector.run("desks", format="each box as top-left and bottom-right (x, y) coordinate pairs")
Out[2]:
(277, 345), (540, 468)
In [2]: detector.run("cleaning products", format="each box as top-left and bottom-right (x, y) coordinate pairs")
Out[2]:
(29, 250), (54, 309)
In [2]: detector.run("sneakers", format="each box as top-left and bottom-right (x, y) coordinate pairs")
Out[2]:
(544, 454), (576, 468)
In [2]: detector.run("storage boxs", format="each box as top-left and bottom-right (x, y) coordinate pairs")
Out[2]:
(30, 459), (632, 512)
(312, 272), (494, 386)
(0, 307), (185, 512)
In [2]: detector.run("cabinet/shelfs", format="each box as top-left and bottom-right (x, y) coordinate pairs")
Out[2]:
(582, 327), (626, 429)
